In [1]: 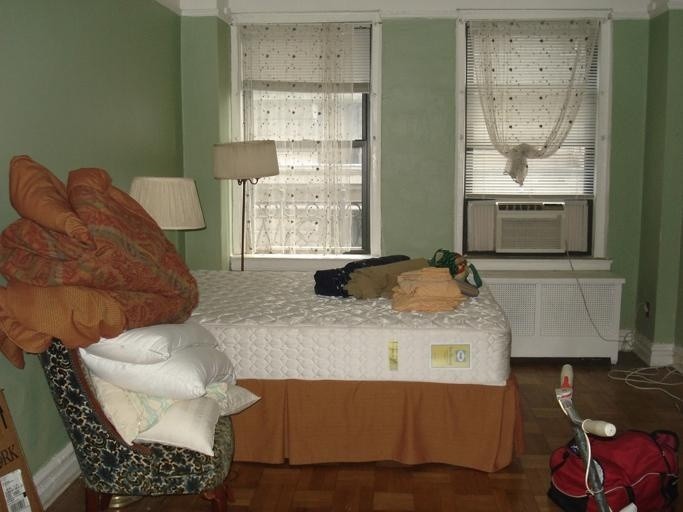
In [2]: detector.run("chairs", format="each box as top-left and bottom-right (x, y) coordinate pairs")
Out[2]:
(35, 335), (237, 510)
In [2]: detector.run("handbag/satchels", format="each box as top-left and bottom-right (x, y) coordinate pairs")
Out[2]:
(429, 249), (470, 282)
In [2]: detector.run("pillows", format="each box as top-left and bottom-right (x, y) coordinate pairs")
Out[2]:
(78, 323), (263, 461)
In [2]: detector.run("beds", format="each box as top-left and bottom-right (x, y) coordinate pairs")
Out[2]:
(185, 266), (528, 475)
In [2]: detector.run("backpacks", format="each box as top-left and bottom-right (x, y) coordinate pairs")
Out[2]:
(548, 430), (680, 512)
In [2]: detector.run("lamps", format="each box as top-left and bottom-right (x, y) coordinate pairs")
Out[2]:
(128, 177), (208, 231)
(212, 140), (280, 270)
